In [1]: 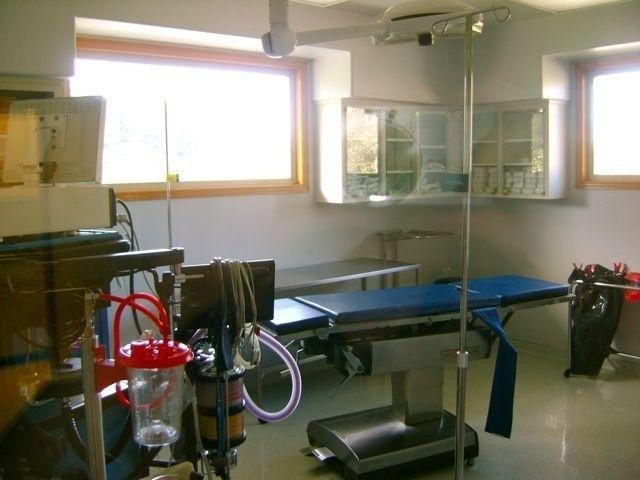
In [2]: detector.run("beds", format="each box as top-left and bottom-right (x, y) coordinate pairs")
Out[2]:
(259, 258), (422, 380)
(258, 274), (576, 480)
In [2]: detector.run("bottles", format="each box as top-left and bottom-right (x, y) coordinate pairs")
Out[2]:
(117, 336), (194, 446)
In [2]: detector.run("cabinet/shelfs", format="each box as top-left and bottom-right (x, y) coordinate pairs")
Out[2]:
(381, 229), (453, 291)
(563, 276), (639, 379)
(310, 96), (571, 204)
